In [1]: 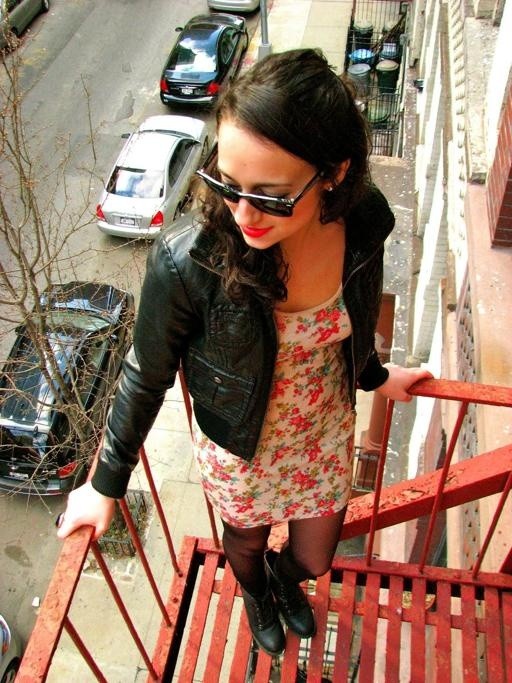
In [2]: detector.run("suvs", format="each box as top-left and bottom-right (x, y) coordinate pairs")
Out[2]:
(1, 280), (137, 500)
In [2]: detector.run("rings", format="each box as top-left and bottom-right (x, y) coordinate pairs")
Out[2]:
(55, 512), (65, 528)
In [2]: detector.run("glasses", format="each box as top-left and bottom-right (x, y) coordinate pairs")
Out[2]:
(195, 140), (329, 218)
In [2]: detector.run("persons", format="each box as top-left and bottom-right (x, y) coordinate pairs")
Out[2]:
(53, 47), (434, 657)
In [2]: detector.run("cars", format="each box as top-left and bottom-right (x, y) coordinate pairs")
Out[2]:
(94, 110), (207, 247)
(159, 9), (249, 110)
(202, 0), (268, 16)
(0, 616), (28, 676)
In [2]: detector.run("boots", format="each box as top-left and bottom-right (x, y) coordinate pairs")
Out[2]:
(264, 550), (316, 639)
(239, 570), (287, 655)
